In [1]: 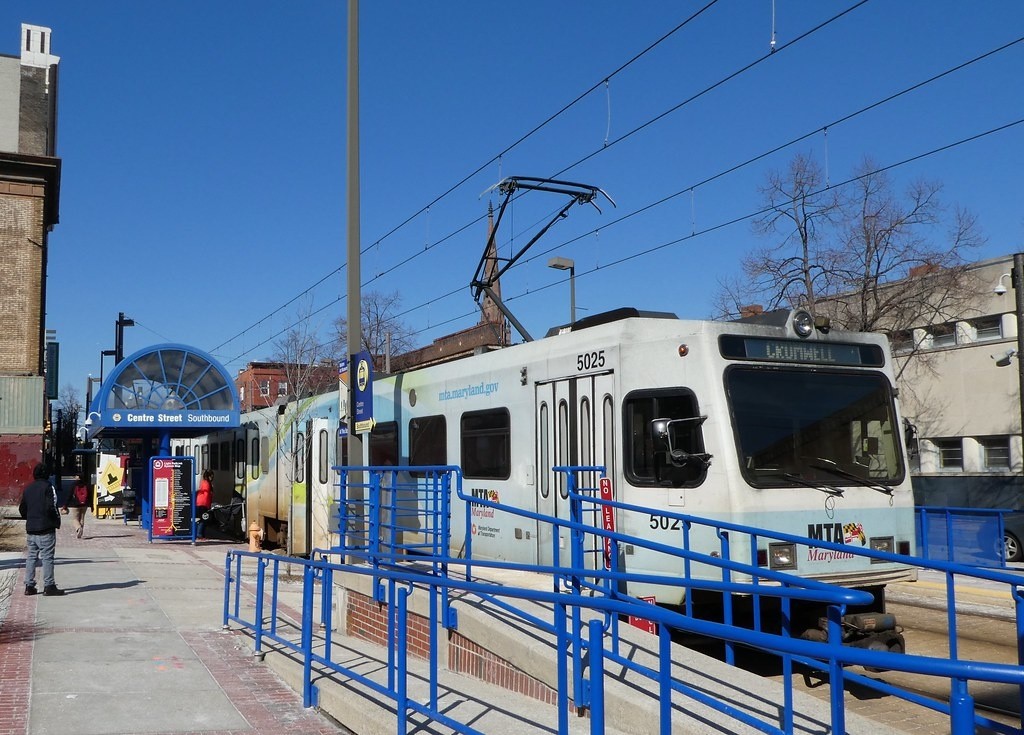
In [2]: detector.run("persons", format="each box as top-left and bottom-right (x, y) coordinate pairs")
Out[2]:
(73, 474), (89, 538)
(19, 465), (65, 596)
(194, 470), (213, 542)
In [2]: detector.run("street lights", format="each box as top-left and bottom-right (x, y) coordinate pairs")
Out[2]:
(114, 319), (135, 368)
(101, 349), (115, 386)
(90, 377), (104, 403)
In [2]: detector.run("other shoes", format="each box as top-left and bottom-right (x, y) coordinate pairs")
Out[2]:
(76, 528), (82, 538)
(196, 537), (209, 541)
(25, 586), (38, 594)
(44, 585), (65, 596)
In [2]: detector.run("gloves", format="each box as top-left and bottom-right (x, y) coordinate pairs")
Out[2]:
(63, 504), (67, 510)
(91, 505), (94, 512)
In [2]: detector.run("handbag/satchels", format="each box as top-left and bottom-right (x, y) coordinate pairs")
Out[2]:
(61, 509), (69, 514)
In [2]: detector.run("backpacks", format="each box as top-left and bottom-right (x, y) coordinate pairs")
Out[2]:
(75, 485), (88, 504)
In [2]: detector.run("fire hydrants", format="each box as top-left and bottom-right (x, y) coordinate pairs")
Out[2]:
(247, 520), (266, 554)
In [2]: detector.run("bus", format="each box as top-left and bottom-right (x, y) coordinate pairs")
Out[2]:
(168, 171), (924, 651)
